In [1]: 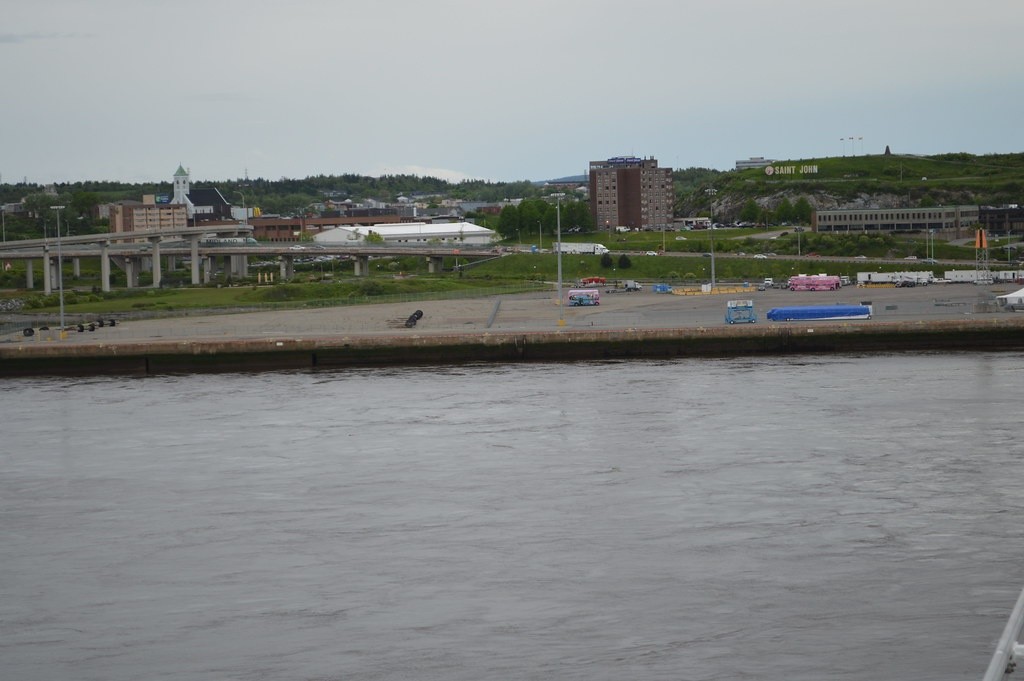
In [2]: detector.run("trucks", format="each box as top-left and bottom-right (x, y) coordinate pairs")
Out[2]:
(652, 284), (672, 294)
(764, 278), (774, 287)
(840, 276), (854, 286)
(491, 246), (521, 253)
(555, 242), (610, 255)
(622, 280), (643, 292)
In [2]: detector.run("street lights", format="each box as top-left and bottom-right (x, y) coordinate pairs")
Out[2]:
(551, 193), (567, 321)
(705, 188), (718, 288)
(51, 205), (66, 331)
(537, 221), (542, 250)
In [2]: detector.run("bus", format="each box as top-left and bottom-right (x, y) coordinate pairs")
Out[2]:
(568, 290), (601, 306)
(790, 275), (841, 291)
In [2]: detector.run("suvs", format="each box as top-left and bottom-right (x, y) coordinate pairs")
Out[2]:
(794, 226), (804, 233)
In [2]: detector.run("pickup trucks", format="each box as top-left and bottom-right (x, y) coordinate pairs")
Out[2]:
(933, 278), (951, 285)
(290, 245), (305, 250)
(310, 246), (326, 250)
(922, 259), (937, 264)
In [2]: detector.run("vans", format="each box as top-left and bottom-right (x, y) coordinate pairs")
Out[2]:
(974, 278), (993, 286)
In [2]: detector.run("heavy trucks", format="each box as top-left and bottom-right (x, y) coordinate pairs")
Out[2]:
(200, 237), (259, 247)
(856, 272), (934, 286)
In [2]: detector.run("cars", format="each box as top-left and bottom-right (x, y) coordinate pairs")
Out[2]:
(703, 253), (712, 257)
(854, 255), (866, 259)
(754, 254), (767, 259)
(758, 284), (765, 291)
(781, 284), (787, 289)
(735, 251), (746, 256)
(904, 256), (917, 261)
(725, 300), (756, 323)
(805, 252), (821, 257)
(645, 251), (658, 256)
(1010, 260), (1022, 266)
(772, 283), (780, 289)
